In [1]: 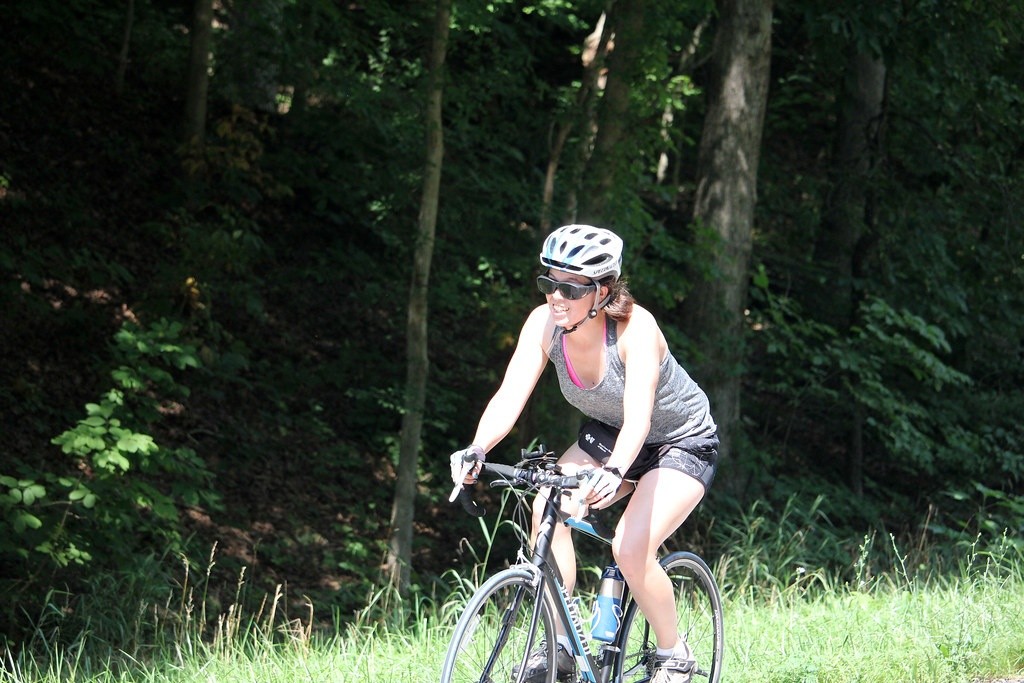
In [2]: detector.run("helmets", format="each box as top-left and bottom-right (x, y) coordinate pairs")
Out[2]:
(541, 225), (625, 284)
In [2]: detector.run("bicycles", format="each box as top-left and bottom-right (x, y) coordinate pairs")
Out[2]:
(441, 444), (723, 683)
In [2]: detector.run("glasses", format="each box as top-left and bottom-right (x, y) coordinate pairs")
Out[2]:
(534, 270), (607, 301)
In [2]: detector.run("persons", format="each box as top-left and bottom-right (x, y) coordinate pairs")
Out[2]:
(449, 224), (719, 683)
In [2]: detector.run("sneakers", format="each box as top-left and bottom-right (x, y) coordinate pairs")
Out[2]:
(647, 642), (696, 683)
(512, 639), (582, 683)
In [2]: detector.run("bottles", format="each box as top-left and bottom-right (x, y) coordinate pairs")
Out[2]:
(590, 558), (624, 641)
